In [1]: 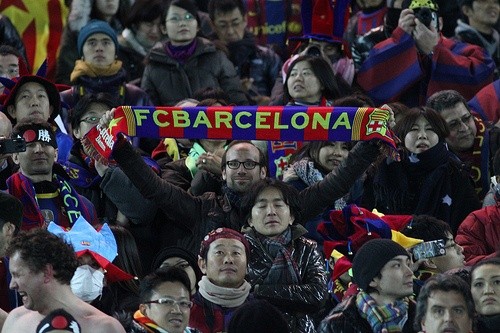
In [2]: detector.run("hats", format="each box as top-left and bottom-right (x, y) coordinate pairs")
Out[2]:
(287, 0), (350, 44)
(0, 75), (61, 123)
(353, 238), (411, 289)
(402, 0), (441, 13)
(78, 20), (118, 58)
(200, 228), (249, 258)
(47, 214), (138, 281)
(151, 246), (201, 287)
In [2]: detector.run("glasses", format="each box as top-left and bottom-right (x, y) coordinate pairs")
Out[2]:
(225, 160), (260, 170)
(443, 241), (456, 249)
(79, 116), (100, 125)
(448, 114), (472, 131)
(144, 298), (192, 311)
(165, 14), (196, 23)
(0, 69), (20, 77)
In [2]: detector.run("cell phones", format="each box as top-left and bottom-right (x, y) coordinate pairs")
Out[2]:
(0, 138), (26, 154)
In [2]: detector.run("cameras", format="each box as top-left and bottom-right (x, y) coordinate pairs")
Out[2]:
(412, 7), (433, 28)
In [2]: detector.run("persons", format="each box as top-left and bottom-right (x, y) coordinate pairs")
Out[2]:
(0, 0), (500, 333)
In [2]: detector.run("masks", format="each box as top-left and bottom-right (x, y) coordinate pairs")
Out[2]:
(70, 264), (103, 302)
